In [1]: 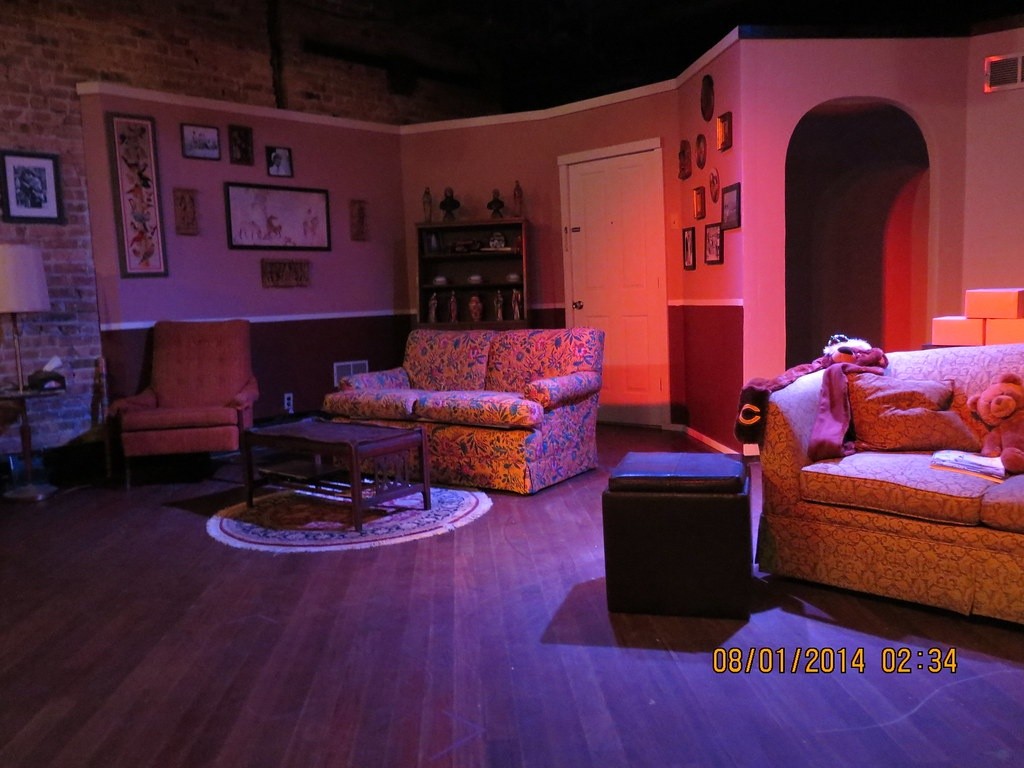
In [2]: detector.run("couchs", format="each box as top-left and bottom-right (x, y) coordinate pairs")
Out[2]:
(319, 327), (605, 496)
(757, 338), (1024, 628)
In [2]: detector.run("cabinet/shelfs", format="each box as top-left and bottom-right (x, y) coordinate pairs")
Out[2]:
(414, 216), (535, 328)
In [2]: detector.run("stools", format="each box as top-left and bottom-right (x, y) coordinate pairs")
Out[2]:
(601, 451), (754, 619)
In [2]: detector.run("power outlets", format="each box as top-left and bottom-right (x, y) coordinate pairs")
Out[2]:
(283, 393), (294, 410)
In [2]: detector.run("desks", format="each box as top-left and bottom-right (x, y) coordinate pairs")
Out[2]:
(0, 380), (69, 503)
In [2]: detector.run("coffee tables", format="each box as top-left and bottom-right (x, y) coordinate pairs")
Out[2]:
(243, 418), (438, 532)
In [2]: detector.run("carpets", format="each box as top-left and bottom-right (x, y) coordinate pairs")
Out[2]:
(203, 477), (494, 554)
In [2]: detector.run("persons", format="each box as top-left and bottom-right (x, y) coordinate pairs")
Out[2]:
(269, 152), (288, 174)
(512, 288), (521, 321)
(20, 167), (42, 209)
(449, 291), (458, 323)
(493, 290), (504, 321)
(429, 293), (437, 323)
(192, 131), (207, 149)
(512, 180), (522, 216)
(423, 187), (432, 224)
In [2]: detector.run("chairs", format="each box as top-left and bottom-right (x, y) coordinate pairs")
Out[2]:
(114, 315), (259, 492)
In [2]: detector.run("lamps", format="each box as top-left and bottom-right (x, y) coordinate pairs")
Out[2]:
(0, 243), (53, 392)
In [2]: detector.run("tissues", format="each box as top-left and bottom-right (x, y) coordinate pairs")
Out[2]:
(25, 356), (68, 391)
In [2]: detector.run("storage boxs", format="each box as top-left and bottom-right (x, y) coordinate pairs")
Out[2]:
(965, 288), (1024, 319)
(932, 316), (985, 346)
(984, 319), (1024, 345)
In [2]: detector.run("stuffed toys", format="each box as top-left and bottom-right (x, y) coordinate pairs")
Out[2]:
(967, 373), (1024, 474)
(821, 333), (883, 365)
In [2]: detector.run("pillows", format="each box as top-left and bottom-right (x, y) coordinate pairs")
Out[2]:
(845, 369), (982, 452)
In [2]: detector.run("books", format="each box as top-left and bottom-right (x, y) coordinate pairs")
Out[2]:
(930, 450), (1007, 484)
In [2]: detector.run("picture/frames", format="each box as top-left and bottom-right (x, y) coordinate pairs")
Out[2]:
(182, 122), (221, 162)
(223, 180), (333, 254)
(105, 111), (168, 279)
(703, 223), (724, 265)
(716, 111), (733, 151)
(694, 186), (705, 220)
(677, 139), (692, 178)
(349, 198), (376, 241)
(695, 133), (707, 168)
(265, 145), (294, 176)
(0, 148), (69, 224)
(721, 182), (740, 231)
(228, 123), (257, 167)
(683, 225), (696, 270)
